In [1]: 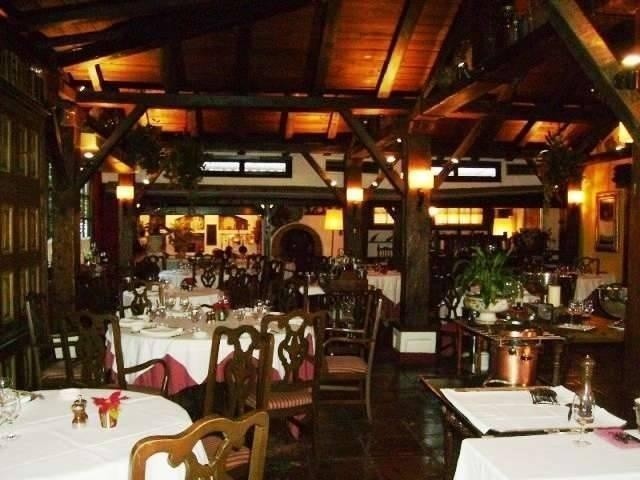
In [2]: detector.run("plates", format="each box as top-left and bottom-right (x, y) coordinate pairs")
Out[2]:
(119, 315), (181, 337)
(558, 322), (597, 333)
(607, 320), (624, 332)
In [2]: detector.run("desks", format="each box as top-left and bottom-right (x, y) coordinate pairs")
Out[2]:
(417, 300), (639, 479)
(573, 270), (616, 300)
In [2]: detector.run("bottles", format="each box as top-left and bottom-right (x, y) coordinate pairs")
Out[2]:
(83, 241), (109, 268)
(571, 353), (599, 427)
(555, 259), (593, 276)
(548, 285), (561, 308)
(71, 399), (88, 426)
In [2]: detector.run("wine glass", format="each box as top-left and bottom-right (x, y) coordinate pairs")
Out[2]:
(502, 269), (558, 323)
(568, 390), (596, 447)
(152, 262), (202, 291)
(566, 297), (594, 324)
(598, 286), (626, 328)
(0, 377), (23, 452)
(158, 294), (275, 330)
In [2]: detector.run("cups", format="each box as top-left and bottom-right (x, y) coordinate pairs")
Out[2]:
(99, 404), (119, 429)
(304, 253), (387, 277)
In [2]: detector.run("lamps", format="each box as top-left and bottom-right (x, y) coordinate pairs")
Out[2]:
(324, 208), (344, 257)
(346, 188), (364, 217)
(567, 189), (584, 218)
(116, 186), (135, 218)
(492, 218), (513, 239)
(407, 169), (434, 211)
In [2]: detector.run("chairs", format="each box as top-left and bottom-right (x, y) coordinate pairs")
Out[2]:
(575, 257), (600, 276)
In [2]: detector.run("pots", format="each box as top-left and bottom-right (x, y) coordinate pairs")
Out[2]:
(490, 341), (542, 386)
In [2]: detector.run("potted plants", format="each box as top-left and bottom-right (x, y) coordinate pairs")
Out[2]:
(450, 244), (524, 324)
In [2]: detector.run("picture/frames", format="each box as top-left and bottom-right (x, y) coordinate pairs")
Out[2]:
(594, 190), (622, 254)
